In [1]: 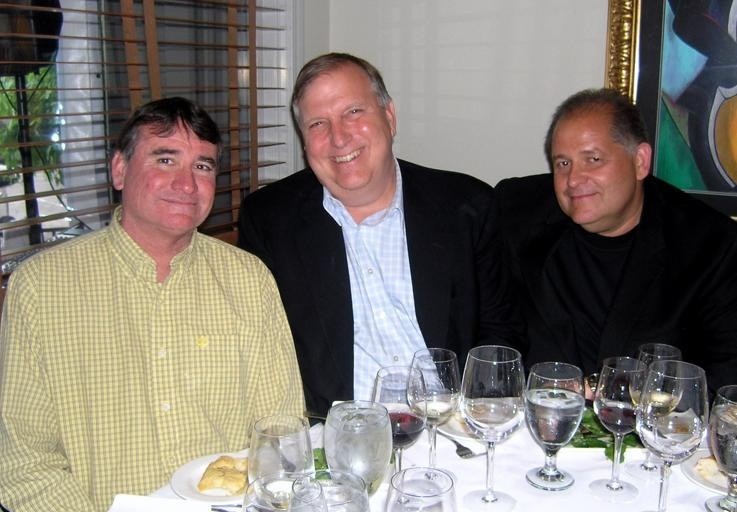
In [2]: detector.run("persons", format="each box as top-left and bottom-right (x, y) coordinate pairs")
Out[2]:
(490, 86), (735, 420)
(0, 95), (312, 512)
(235, 50), (526, 431)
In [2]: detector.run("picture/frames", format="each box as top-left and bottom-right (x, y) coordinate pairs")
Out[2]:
(603, 1), (737, 221)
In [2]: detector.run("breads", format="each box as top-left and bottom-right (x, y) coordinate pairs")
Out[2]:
(198, 455), (248, 494)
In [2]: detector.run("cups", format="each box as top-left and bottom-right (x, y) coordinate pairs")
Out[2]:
(244, 467), (329, 512)
(289, 468), (371, 512)
(247, 414), (315, 479)
(382, 467), (458, 512)
(321, 398), (395, 499)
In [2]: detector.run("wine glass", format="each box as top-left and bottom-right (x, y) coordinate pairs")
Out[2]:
(622, 342), (683, 483)
(460, 344), (525, 512)
(706, 380), (736, 512)
(524, 360), (583, 492)
(405, 347), (462, 471)
(586, 342), (649, 503)
(372, 367), (427, 477)
(634, 359), (710, 512)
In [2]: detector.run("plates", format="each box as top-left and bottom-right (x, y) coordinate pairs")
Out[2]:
(168, 451), (252, 504)
(680, 449), (729, 496)
